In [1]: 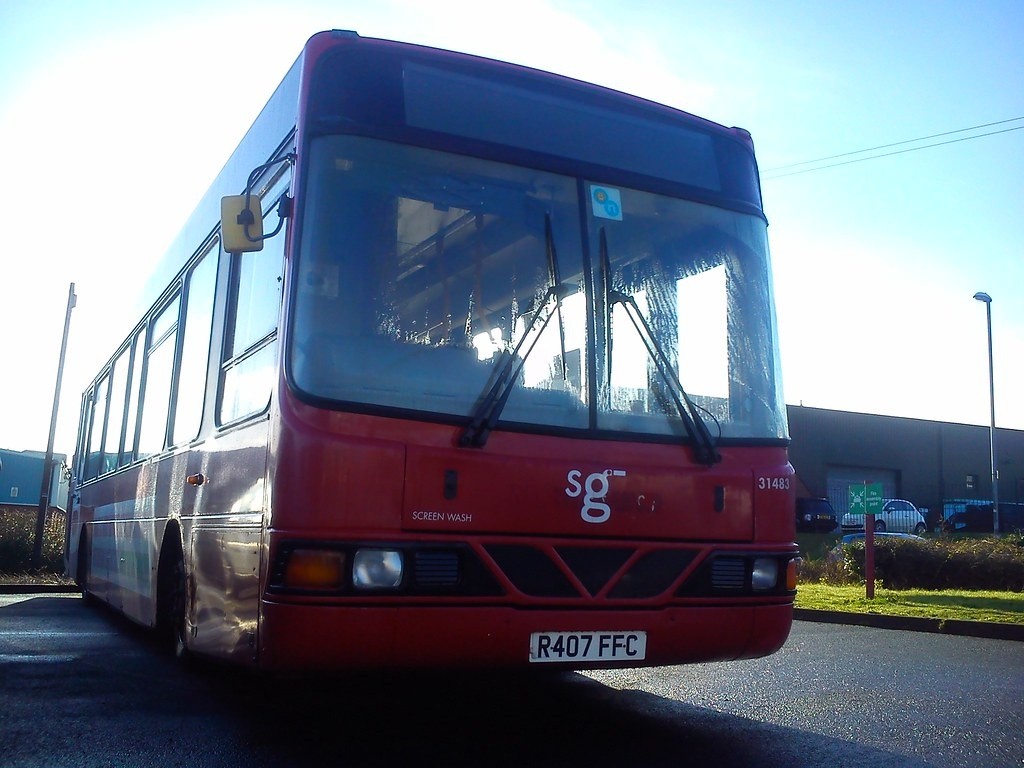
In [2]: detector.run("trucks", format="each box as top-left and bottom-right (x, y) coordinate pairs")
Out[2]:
(942, 499), (994, 529)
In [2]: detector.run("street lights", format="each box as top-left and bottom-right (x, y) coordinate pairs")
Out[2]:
(973, 292), (1001, 537)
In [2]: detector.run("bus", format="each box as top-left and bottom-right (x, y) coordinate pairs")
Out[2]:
(62, 28), (796, 679)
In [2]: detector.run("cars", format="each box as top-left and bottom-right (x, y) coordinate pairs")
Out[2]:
(842, 498), (928, 537)
(795, 497), (840, 534)
(828, 532), (928, 568)
